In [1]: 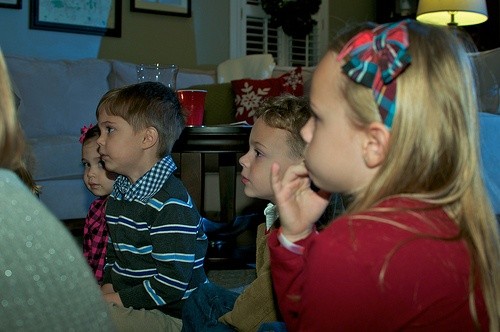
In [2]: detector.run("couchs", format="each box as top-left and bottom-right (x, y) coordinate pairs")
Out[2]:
(6, 58), (315, 222)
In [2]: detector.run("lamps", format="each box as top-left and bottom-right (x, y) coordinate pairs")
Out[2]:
(415, 0), (488, 35)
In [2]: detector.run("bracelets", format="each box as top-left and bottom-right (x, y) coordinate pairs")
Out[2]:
(277, 226), (320, 255)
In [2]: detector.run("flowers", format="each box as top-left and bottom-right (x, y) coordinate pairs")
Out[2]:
(80, 124), (93, 144)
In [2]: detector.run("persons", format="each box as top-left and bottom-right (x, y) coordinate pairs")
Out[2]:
(96, 81), (212, 332)
(79, 122), (116, 288)
(269, 19), (500, 332)
(0, 45), (115, 332)
(181, 94), (341, 332)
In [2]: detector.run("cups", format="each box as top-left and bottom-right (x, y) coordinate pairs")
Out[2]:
(136, 62), (179, 91)
(175, 89), (208, 128)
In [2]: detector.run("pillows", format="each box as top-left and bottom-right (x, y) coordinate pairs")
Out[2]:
(175, 52), (308, 172)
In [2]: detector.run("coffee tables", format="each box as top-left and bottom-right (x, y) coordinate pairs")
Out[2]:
(173, 124), (262, 268)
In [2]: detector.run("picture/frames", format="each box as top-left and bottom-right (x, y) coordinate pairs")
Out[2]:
(0, 0), (123, 38)
(131, 0), (192, 18)
